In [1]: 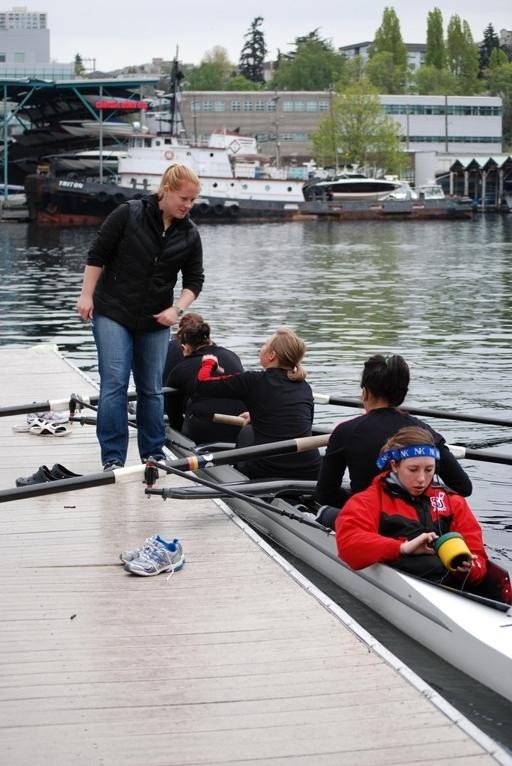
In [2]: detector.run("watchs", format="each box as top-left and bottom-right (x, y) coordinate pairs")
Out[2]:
(172, 303), (184, 317)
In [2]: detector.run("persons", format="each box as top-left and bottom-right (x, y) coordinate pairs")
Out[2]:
(161, 311), (217, 386)
(332, 425), (490, 596)
(192, 325), (322, 479)
(163, 321), (245, 444)
(75, 163), (208, 473)
(315, 350), (473, 531)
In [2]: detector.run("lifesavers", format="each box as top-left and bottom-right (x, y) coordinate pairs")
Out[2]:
(165, 151), (174, 160)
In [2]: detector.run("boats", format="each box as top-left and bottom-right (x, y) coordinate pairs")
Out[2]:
(127, 385), (512, 704)
(377, 182), (473, 214)
(298, 170), (410, 216)
(24, 131), (322, 227)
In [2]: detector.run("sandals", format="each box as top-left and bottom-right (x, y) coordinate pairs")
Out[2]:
(15, 464), (83, 487)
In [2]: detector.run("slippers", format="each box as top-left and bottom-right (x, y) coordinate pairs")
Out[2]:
(13, 418), (69, 437)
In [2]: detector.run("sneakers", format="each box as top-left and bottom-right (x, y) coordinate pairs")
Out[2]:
(120, 534), (185, 576)
(27, 411), (69, 425)
(103, 461), (123, 472)
